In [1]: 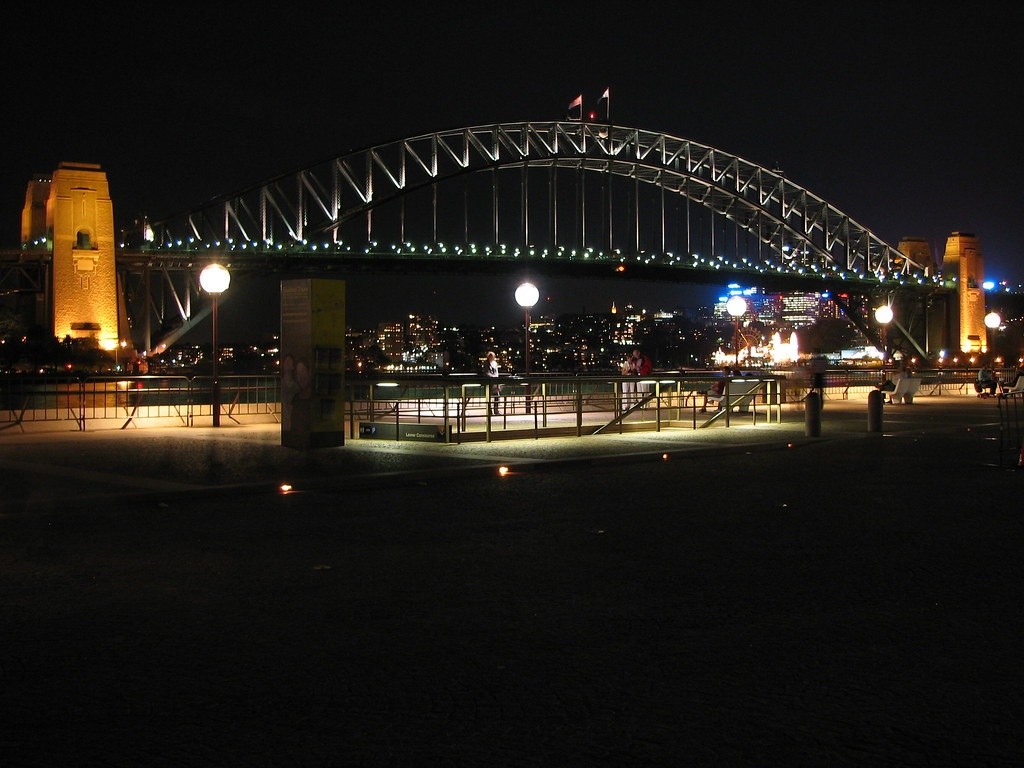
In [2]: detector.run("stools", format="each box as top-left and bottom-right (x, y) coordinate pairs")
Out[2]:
(975, 378), (991, 400)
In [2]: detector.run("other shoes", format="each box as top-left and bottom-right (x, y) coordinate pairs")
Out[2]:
(875, 385), (881, 389)
(697, 391), (707, 394)
(699, 409), (706, 412)
(713, 409), (721, 414)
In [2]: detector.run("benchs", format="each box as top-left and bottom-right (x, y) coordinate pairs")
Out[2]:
(881, 378), (921, 404)
(708, 378), (763, 413)
(1003, 375), (1024, 398)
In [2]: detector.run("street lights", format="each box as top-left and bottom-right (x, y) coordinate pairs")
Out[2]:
(726, 295), (747, 370)
(514, 282), (539, 414)
(874, 305), (893, 380)
(199, 263), (231, 427)
(984, 311), (1001, 371)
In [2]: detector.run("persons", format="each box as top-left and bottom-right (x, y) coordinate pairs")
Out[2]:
(696, 367), (754, 413)
(977, 363), (997, 398)
(1002, 364), (1024, 399)
(482, 351), (503, 416)
(282, 353), (330, 432)
(875, 366), (913, 405)
(811, 347), (828, 409)
(621, 349), (653, 410)
